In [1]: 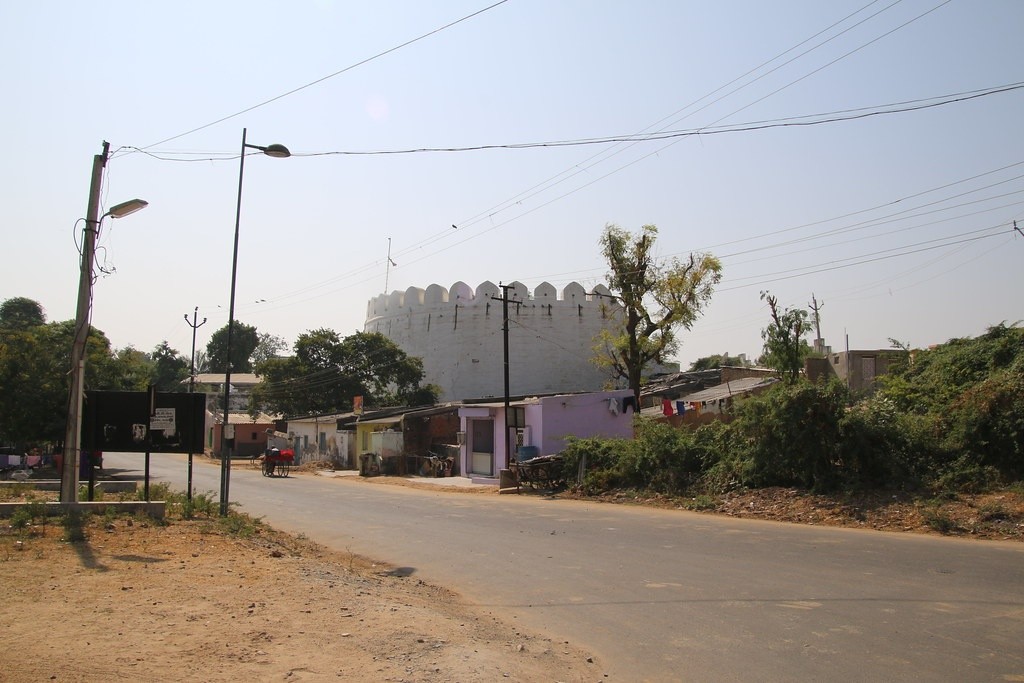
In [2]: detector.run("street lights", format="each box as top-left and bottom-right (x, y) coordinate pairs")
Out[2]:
(218, 127), (293, 517)
(60, 198), (149, 502)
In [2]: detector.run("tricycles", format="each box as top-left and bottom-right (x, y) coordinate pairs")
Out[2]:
(261, 451), (289, 478)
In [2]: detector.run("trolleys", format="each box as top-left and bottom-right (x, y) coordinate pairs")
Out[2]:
(509, 457), (567, 491)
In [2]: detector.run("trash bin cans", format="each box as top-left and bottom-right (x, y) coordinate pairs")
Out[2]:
(359, 453), (374, 476)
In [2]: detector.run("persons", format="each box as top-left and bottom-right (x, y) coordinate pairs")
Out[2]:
(259, 445), (280, 477)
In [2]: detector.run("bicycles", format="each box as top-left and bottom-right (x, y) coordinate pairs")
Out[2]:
(421, 450), (448, 477)
